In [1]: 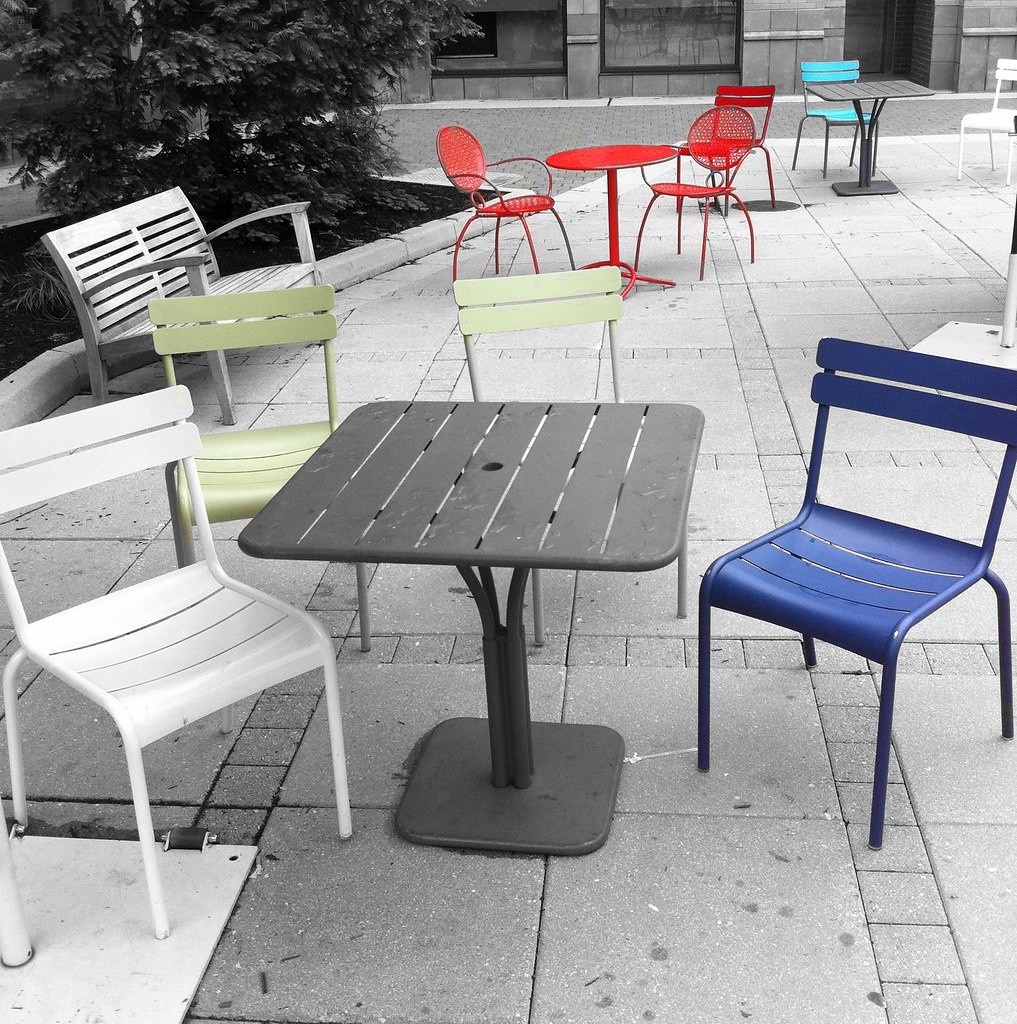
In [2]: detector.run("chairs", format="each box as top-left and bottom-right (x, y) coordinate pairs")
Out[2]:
(0, 385), (356, 940)
(790, 59), (880, 179)
(697, 335), (1017, 852)
(955, 58), (1017, 187)
(633, 105), (756, 281)
(451, 264), (690, 649)
(436, 125), (576, 281)
(147, 282), (370, 654)
(676, 85), (775, 215)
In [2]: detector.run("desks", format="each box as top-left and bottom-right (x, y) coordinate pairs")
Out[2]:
(236, 398), (705, 856)
(806, 79), (935, 198)
(543, 145), (679, 299)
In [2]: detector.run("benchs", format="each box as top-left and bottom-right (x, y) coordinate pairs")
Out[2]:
(40, 184), (323, 428)
